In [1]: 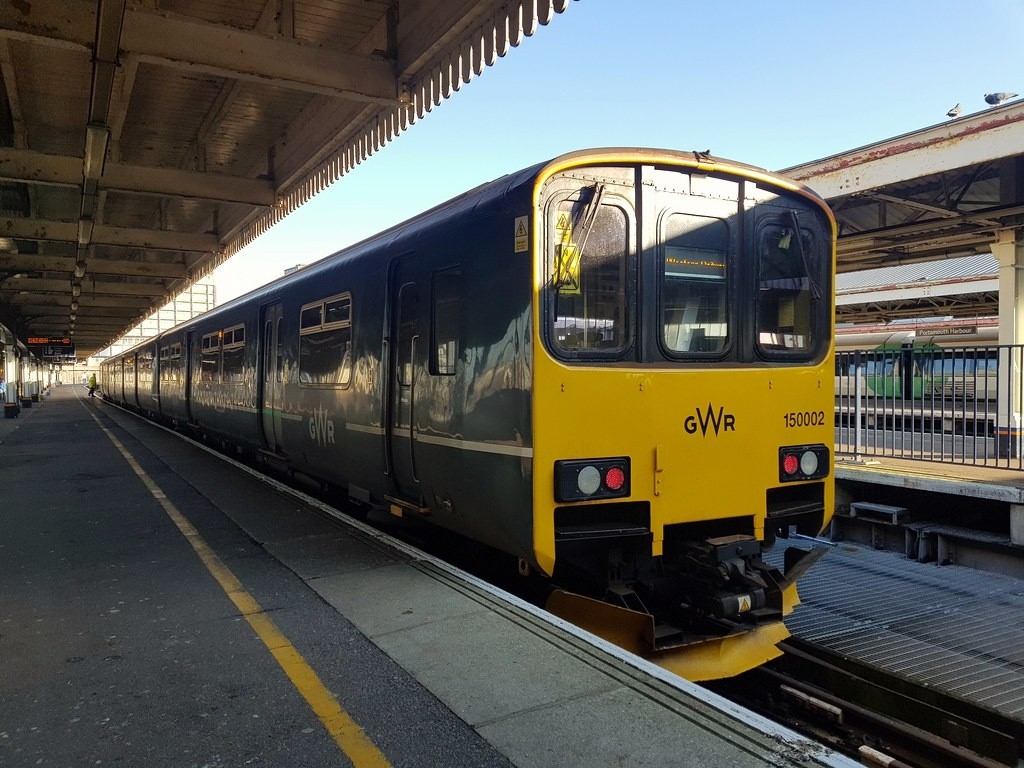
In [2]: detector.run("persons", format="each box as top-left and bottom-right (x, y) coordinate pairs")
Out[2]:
(88, 373), (96, 397)
(0, 379), (6, 401)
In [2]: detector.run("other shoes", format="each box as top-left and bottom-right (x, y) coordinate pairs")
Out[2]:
(92, 395), (96, 397)
(88, 393), (90, 396)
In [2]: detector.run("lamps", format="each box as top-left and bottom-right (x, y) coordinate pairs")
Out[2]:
(77, 217), (93, 245)
(73, 285), (82, 297)
(72, 303), (78, 310)
(84, 121), (109, 179)
(74, 261), (87, 277)
(69, 315), (76, 335)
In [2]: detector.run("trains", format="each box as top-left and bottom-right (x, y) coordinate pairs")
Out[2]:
(99, 146), (836, 683)
(835, 328), (998, 437)
